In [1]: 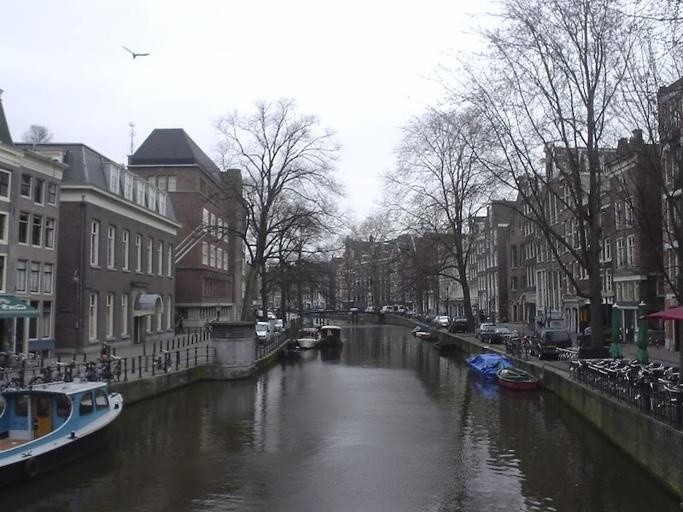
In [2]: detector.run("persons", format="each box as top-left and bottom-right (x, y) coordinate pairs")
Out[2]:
(177, 315), (186, 334)
(101, 352), (119, 368)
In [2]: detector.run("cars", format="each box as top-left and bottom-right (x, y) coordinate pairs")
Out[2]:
(446, 316), (467, 334)
(474, 323), (571, 360)
(364, 304), (449, 327)
(254, 309), (283, 344)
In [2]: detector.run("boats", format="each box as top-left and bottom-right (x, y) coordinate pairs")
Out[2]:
(411, 326), (431, 338)
(320, 325), (342, 348)
(0, 376), (123, 467)
(464, 353), (538, 391)
(295, 328), (321, 349)
